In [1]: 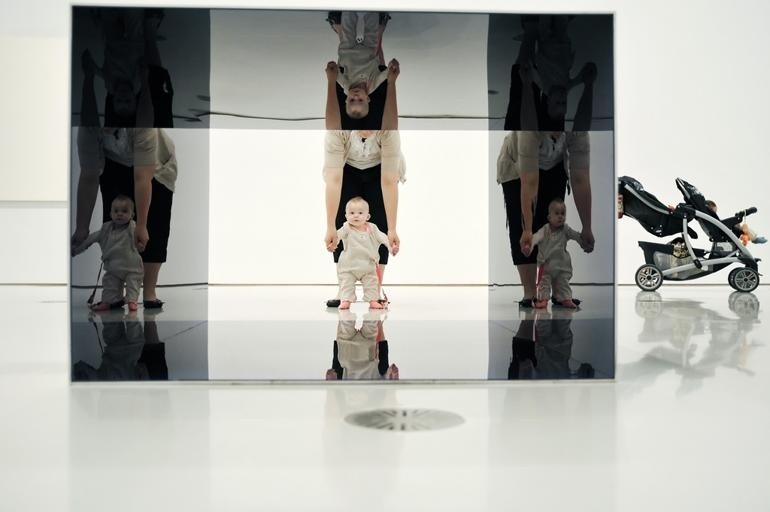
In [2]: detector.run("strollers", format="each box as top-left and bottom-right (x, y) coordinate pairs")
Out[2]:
(618, 176), (768, 292)
(616, 292), (766, 397)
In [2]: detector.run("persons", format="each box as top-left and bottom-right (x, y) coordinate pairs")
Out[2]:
(506, 307), (595, 379)
(77, 8), (176, 127)
(70, 127), (181, 309)
(324, 10), (400, 130)
(518, 13), (596, 119)
(518, 309), (582, 379)
(336, 310), (394, 381)
(326, 310), (400, 380)
(320, 129), (407, 306)
(72, 193), (151, 312)
(72, 309), (170, 381)
(96, 8), (155, 118)
(328, 11), (396, 120)
(502, 13), (597, 130)
(702, 199), (769, 245)
(95, 310), (147, 378)
(326, 196), (400, 311)
(494, 131), (596, 309)
(521, 197), (593, 310)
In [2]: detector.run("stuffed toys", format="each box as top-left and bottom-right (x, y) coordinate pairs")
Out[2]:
(738, 222), (752, 246)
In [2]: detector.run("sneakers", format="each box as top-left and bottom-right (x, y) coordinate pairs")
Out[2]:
(752, 235), (769, 244)
(520, 295), (580, 308)
(326, 298), (390, 310)
(90, 297), (164, 310)
